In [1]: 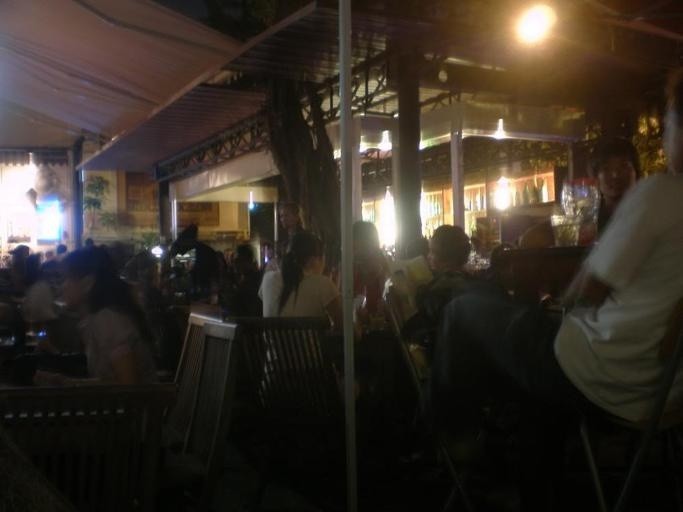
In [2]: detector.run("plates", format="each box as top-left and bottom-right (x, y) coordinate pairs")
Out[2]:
(362, 165), (567, 248)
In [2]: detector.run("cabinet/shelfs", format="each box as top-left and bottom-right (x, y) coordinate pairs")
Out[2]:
(362, 165), (567, 248)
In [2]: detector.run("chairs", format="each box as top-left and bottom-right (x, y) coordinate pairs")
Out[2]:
(0, 277), (683, 512)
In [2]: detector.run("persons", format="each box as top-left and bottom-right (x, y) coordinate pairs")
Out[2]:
(476, 78), (682, 512)
(110, 227), (258, 364)
(1, 238), (97, 348)
(587, 136), (640, 243)
(36, 246), (159, 511)
(353, 215), (571, 507)
(257, 234), (366, 406)
